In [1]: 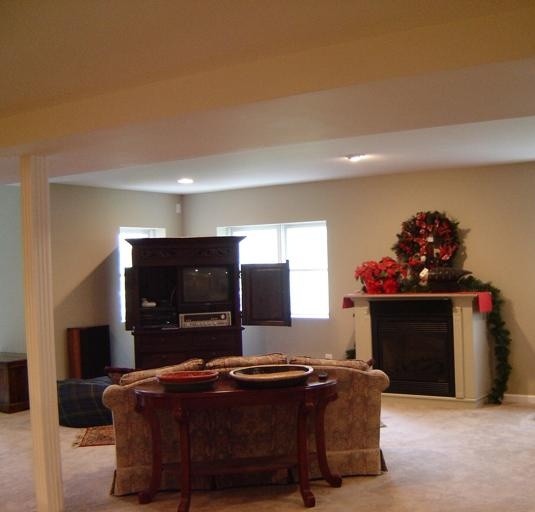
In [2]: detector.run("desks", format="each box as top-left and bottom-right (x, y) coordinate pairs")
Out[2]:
(0, 352), (29, 413)
(133, 375), (342, 512)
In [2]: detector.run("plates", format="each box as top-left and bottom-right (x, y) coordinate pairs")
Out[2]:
(156, 370), (220, 392)
(229, 364), (314, 386)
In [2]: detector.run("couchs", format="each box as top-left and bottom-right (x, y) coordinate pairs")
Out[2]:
(103, 353), (390, 494)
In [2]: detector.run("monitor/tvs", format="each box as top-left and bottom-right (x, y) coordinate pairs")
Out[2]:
(176, 265), (234, 312)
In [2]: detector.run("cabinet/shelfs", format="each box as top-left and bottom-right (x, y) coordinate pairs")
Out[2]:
(342, 292), (494, 403)
(125, 236), (291, 369)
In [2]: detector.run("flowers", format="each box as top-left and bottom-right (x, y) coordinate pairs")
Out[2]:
(391, 210), (460, 270)
(355, 255), (409, 281)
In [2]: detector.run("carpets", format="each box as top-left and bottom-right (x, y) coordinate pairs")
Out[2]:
(73, 425), (115, 447)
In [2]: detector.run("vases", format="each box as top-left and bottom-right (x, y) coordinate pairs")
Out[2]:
(364, 278), (399, 294)
(409, 265), (442, 291)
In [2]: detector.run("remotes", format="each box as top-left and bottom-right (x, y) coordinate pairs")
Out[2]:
(319, 371), (328, 380)
